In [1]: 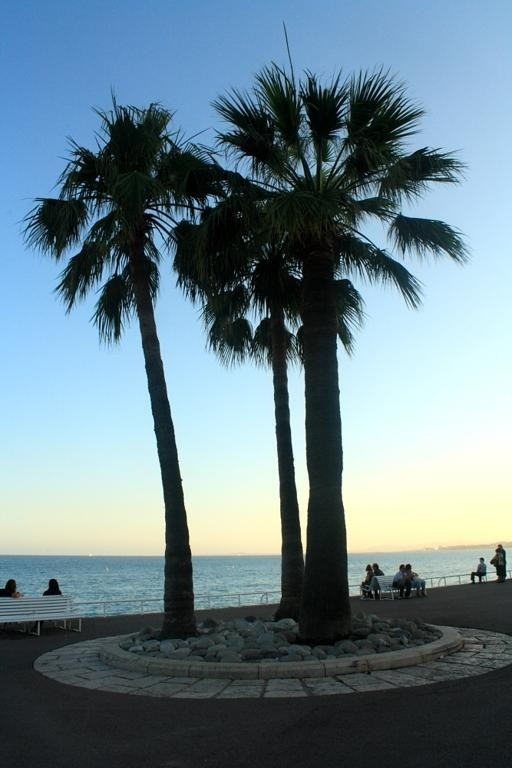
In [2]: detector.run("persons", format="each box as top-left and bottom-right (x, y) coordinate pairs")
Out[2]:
(498, 544), (507, 582)
(0, 579), (17, 632)
(31, 579), (62, 632)
(471, 558), (486, 584)
(490, 548), (504, 583)
(405, 564), (427, 597)
(373, 563), (385, 599)
(362, 565), (379, 599)
(392, 565), (412, 599)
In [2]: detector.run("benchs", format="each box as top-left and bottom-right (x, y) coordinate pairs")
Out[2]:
(376, 576), (417, 600)
(0, 594), (82, 636)
(360, 576), (376, 601)
